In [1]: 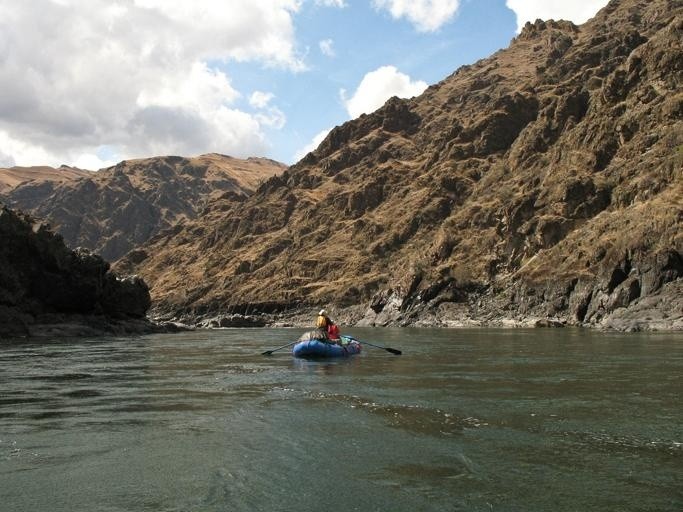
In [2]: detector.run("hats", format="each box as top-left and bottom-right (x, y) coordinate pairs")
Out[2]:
(319, 310), (326, 315)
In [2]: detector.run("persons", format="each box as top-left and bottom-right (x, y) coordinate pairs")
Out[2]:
(317, 309), (333, 334)
(328, 320), (340, 339)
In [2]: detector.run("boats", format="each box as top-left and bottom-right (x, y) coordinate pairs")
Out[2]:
(293, 336), (361, 357)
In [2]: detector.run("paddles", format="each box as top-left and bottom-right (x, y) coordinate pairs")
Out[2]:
(344, 336), (402, 354)
(261, 339), (301, 355)
